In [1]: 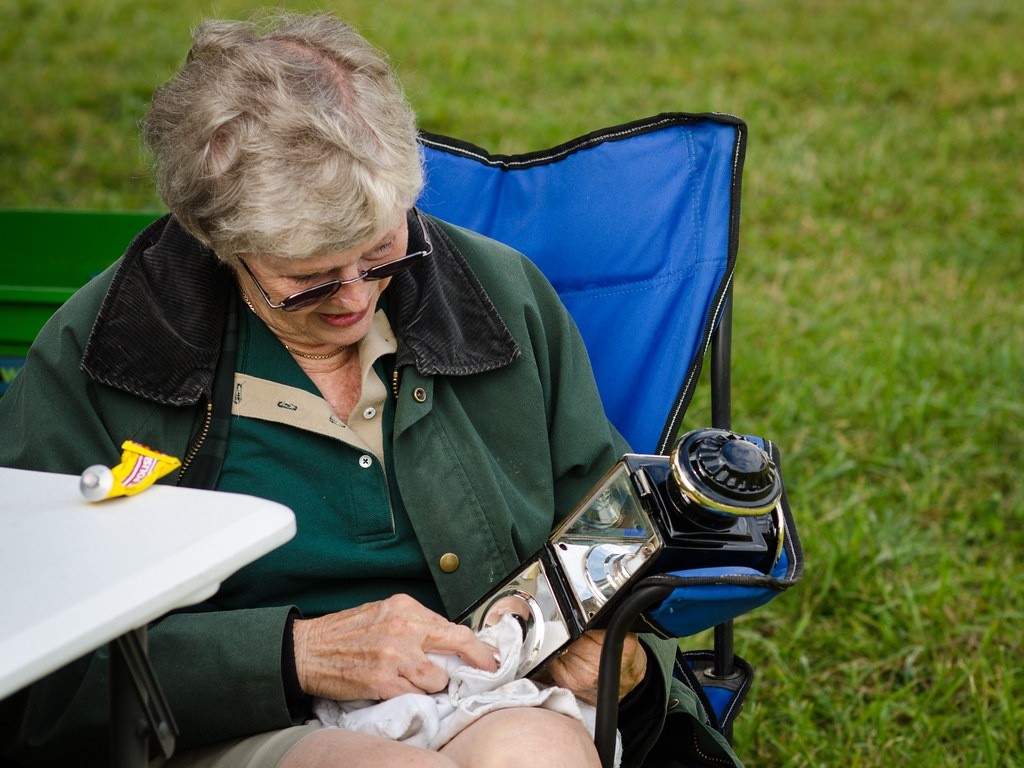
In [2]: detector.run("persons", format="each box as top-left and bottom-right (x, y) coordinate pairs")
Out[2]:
(0, 11), (680, 767)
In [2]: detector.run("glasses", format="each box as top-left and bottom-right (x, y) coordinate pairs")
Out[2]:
(233, 202), (434, 312)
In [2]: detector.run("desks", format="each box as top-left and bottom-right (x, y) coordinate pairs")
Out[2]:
(0, 465), (297, 768)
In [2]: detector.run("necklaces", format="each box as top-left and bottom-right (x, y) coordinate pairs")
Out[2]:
(236, 281), (349, 360)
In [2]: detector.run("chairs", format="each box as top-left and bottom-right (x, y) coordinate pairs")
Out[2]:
(410, 112), (800, 768)
